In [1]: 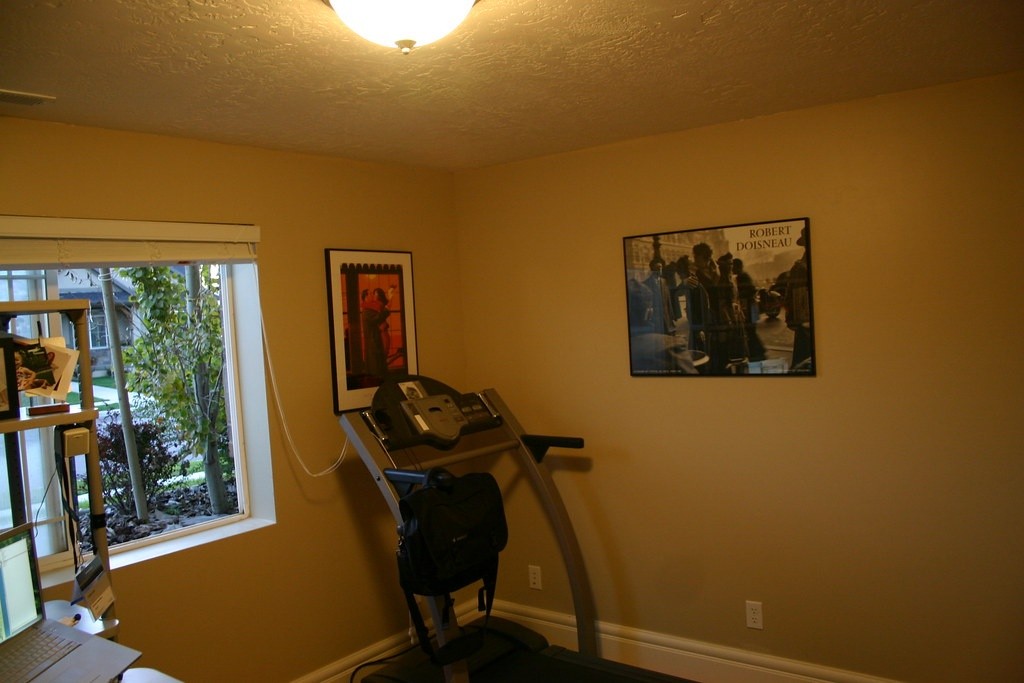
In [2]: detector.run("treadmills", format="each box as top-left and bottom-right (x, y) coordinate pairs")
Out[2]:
(341, 374), (695, 683)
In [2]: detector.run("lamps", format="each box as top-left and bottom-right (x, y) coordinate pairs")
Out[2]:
(321, 0), (480, 56)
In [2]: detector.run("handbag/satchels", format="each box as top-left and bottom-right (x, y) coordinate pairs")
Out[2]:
(395, 466), (509, 664)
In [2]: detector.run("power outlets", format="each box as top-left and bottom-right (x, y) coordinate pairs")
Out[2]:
(528, 563), (543, 592)
(745, 600), (764, 630)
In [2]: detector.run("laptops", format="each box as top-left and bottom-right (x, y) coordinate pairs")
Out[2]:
(0, 522), (142, 683)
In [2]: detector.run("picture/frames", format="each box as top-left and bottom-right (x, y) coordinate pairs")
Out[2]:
(621, 217), (819, 382)
(325, 248), (420, 414)
(0, 338), (21, 420)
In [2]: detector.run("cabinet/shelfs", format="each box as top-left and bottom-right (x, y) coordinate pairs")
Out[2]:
(0, 299), (120, 641)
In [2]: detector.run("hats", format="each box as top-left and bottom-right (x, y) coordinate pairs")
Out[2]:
(796, 227), (806, 246)
(628, 279), (653, 309)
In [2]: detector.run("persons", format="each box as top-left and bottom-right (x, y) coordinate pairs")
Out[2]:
(626, 228), (815, 374)
(357, 285), (394, 377)
